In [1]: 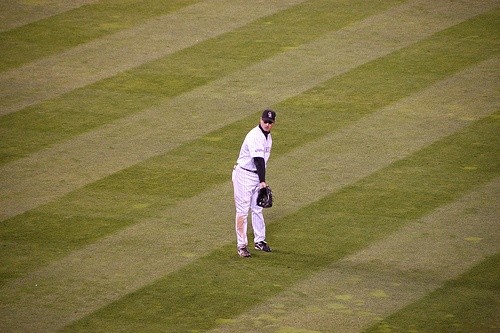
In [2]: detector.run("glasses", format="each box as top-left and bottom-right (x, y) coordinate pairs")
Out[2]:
(262, 119), (272, 123)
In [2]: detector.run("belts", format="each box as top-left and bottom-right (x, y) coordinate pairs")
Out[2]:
(240, 166), (258, 174)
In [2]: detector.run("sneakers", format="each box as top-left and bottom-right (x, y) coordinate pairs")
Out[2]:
(254, 240), (272, 252)
(237, 248), (251, 256)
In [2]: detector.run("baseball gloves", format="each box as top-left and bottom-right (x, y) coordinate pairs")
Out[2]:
(256, 187), (272, 208)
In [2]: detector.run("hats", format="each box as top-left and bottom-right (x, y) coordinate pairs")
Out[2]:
(262, 109), (276, 123)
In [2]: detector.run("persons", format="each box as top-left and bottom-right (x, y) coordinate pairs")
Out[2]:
(230, 109), (282, 256)
